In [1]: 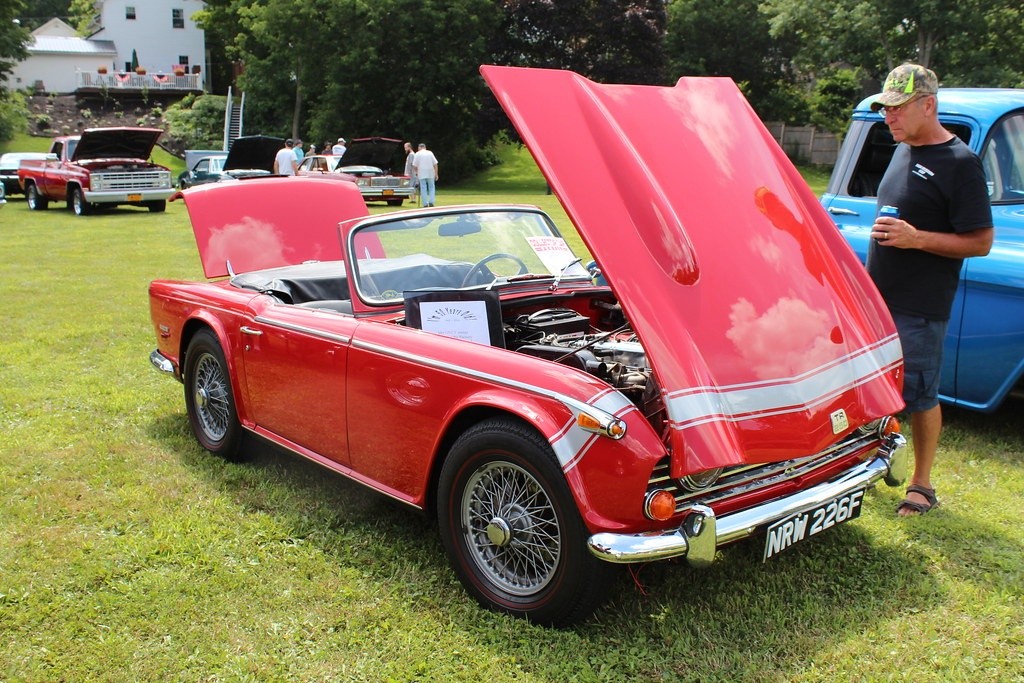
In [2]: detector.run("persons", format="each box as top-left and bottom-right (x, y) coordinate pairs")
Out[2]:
(864, 62), (995, 517)
(404, 142), (417, 203)
(331, 137), (346, 155)
(274, 139), (299, 175)
(293, 140), (304, 164)
(305, 144), (316, 166)
(411, 143), (438, 208)
(322, 140), (333, 155)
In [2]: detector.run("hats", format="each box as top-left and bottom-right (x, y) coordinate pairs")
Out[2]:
(871, 61), (938, 112)
(309, 144), (316, 148)
(296, 139), (303, 145)
(338, 138), (346, 143)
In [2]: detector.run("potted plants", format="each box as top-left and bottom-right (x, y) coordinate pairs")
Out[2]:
(174, 68), (185, 76)
(98, 65), (107, 74)
(135, 66), (147, 75)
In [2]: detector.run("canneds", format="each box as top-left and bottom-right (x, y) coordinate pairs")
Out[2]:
(874, 206), (900, 241)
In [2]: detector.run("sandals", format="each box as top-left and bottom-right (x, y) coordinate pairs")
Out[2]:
(896, 485), (936, 516)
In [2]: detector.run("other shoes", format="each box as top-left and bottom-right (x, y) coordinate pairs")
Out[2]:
(409, 201), (416, 203)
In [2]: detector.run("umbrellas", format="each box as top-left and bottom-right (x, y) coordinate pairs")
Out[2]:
(132, 49), (138, 72)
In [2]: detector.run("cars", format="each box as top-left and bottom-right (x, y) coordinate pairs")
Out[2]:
(149, 69), (906, 624)
(177, 134), (287, 190)
(298, 136), (416, 208)
(0, 152), (47, 204)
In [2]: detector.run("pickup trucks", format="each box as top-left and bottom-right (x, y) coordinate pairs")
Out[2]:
(586, 85), (1024, 420)
(17, 125), (176, 216)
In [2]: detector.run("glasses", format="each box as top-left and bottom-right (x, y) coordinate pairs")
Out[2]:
(878, 95), (927, 118)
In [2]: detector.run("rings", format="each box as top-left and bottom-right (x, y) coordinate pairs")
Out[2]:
(885, 233), (887, 239)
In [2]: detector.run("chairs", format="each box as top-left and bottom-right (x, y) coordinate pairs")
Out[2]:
(857, 165), (886, 197)
